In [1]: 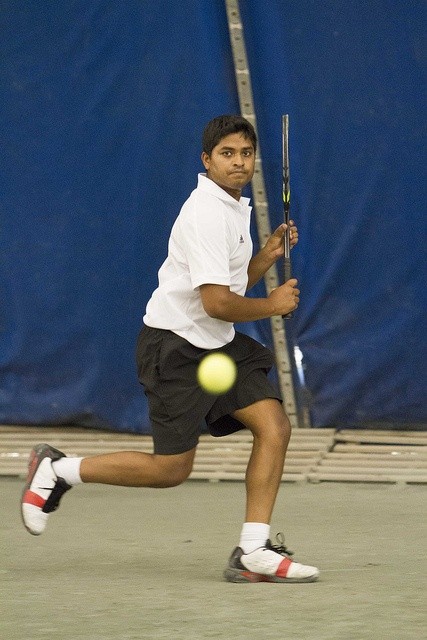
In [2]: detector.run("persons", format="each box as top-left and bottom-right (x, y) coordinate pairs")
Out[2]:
(20, 114), (319, 583)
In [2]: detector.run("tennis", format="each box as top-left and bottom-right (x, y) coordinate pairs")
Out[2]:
(195, 353), (238, 393)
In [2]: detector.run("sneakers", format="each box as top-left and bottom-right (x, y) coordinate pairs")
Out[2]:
(20, 443), (72, 536)
(224, 532), (319, 583)
